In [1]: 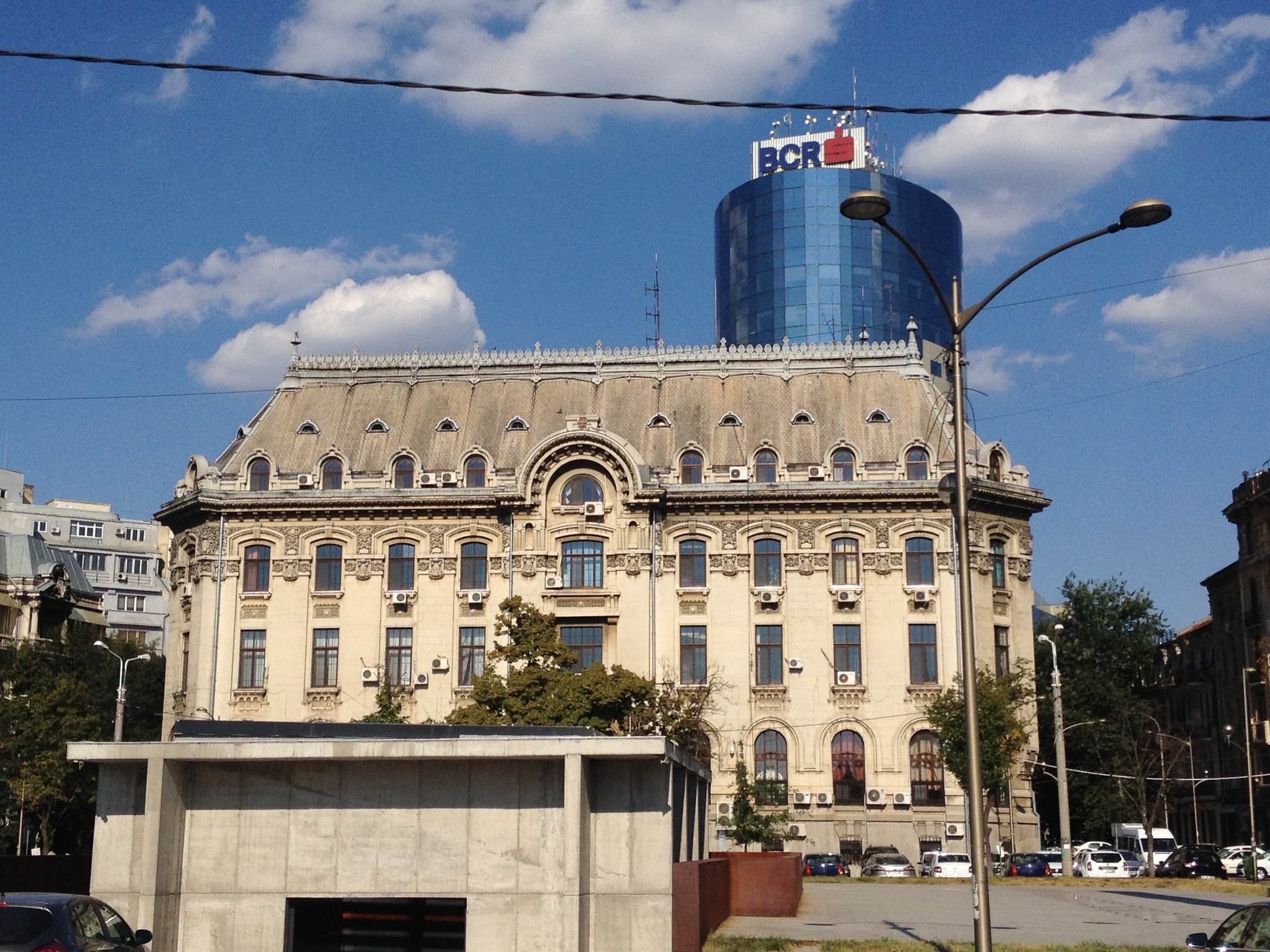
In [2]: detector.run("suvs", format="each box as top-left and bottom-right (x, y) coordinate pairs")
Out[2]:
(859, 844), (900, 870)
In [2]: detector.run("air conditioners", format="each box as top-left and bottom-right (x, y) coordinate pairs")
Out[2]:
(362, 668), (380, 682)
(716, 787), (966, 839)
(545, 576), (562, 588)
(414, 657), (448, 685)
(392, 593), (406, 603)
(761, 590), (930, 603)
(298, 474), (314, 486)
(117, 573), (127, 580)
(584, 502), (603, 515)
(115, 528), (123, 534)
(52, 527), (59, 533)
(837, 671), (855, 685)
(808, 466), (824, 478)
(729, 467), (748, 480)
(789, 658), (803, 668)
(468, 592), (482, 603)
(420, 472), (457, 484)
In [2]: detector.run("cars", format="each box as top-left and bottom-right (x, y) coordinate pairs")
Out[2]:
(1179, 843), (1270, 880)
(1001, 853), (1053, 877)
(929, 853), (973, 878)
(1034, 850), (1063, 877)
(801, 853), (851, 877)
(1154, 849), (1228, 881)
(1072, 849), (1145, 878)
(1055, 840), (1085, 851)
(1071, 841), (1113, 858)
(1186, 900), (1270, 952)
(917, 851), (947, 877)
(861, 853), (916, 878)
(983, 850), (1010, 876)
(0, 892), (156, 952)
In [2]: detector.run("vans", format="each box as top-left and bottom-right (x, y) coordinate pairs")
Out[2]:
(1120, 827), (1179, 868)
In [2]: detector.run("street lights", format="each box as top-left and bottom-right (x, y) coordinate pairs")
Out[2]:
(836, 190), (1173, 952)
(1007, 634), (1106, 873)
(93, 640), (151, 741)
(1129, 666), (1266, 882)
